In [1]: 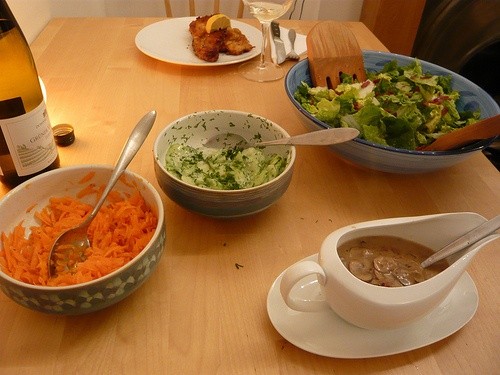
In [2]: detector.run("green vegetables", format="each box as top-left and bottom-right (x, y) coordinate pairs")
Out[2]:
(295, 59), (482, 153)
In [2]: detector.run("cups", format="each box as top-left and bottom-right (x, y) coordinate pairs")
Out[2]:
(280, 210), (500, 331)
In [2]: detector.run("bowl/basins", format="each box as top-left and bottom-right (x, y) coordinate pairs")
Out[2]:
(153, 109), (296, 216)
(0, 164), (167, 318)
(286, 50), (500, 175)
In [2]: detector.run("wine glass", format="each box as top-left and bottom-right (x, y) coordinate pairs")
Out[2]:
(239, 0), (292, 83)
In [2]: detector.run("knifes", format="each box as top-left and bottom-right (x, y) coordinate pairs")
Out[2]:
(271, 20), (286, 65)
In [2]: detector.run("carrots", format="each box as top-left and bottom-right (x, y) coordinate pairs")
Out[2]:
(1, 172), (157, 288)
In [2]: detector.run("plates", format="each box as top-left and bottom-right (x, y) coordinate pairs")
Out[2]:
(266, 252), (479, 358)
(133, 16), (267, 68)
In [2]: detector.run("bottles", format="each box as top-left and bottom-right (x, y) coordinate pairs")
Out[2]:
(0, 0), (60, 188)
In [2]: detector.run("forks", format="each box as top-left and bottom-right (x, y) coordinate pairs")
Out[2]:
(286, 28), (299, 61)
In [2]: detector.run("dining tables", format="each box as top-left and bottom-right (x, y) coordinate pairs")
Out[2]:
(0, 15), (500, 374)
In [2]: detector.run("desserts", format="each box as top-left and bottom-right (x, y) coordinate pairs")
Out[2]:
(189, 14), (254, 62)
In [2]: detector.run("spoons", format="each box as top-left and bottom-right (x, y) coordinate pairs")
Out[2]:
(420, 214), (500, 267)
(47, 107), (157, 281)
(202, 126), (361, 153)
